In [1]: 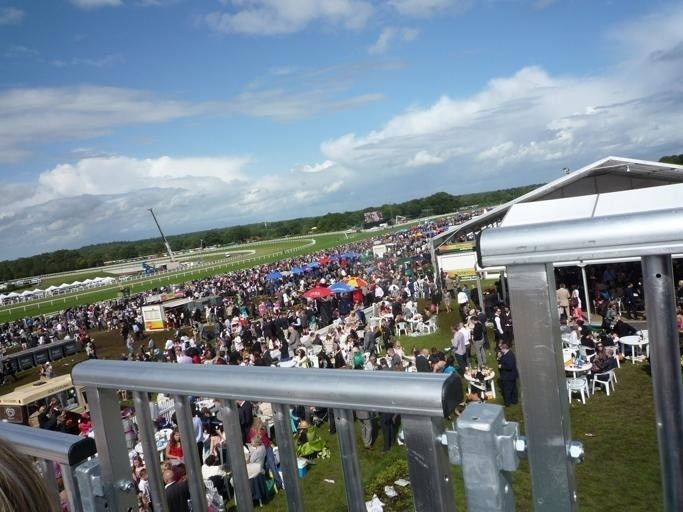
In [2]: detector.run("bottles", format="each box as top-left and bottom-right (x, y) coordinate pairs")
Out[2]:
(464, 363), (493, 379)
(411, 345), (416, 356)
(570, 353), (587, 368)
(639, 329), (643, 340)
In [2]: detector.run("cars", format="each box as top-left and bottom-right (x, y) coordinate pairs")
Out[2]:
(223, 251), (230, 258)
(401, 220), (449, 247)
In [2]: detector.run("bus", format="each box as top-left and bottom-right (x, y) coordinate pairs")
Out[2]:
(0, 373), (89, 429)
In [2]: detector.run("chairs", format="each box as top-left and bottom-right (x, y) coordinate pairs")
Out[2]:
(87, 297), (345, 511)
(345, 299), (650, 410)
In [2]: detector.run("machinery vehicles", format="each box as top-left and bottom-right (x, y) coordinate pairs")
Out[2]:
(145, 207), (183, 271)
